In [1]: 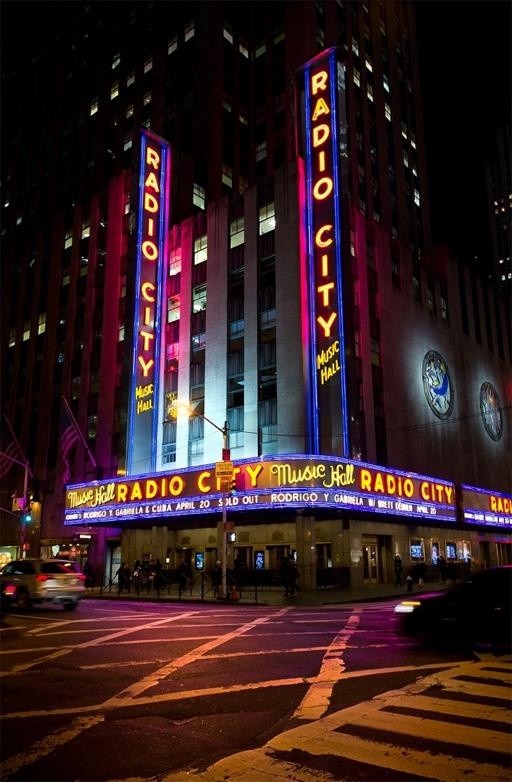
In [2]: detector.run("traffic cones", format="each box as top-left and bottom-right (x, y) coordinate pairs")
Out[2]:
(231, 583), (240, 601)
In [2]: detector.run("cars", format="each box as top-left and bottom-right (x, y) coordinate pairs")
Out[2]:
(390, 565), (512, 651)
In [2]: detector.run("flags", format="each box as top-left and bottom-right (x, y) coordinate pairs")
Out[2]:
(58, 405), (80, 484)
(0, 419), (21, 479)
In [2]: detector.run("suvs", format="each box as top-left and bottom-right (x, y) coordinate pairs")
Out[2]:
(0, 559), (87, 610)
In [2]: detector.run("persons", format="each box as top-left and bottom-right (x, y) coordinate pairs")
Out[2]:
(211, 560), (222, 592)
(436, 555), (447, 584)
(115, 562), (130, 595)
(129, 559), (148, 595)
(177, 559), (193, 593)
(448, 558), (457, 585)
(416, 559), (425, 589)
(278, 556), (300, 597)
(149, 558), (162, 592)
(393, 555), (403, 587)
(288, 553), (298, 568)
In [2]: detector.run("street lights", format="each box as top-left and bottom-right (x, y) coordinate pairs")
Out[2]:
(168, 401), (229, 600)
